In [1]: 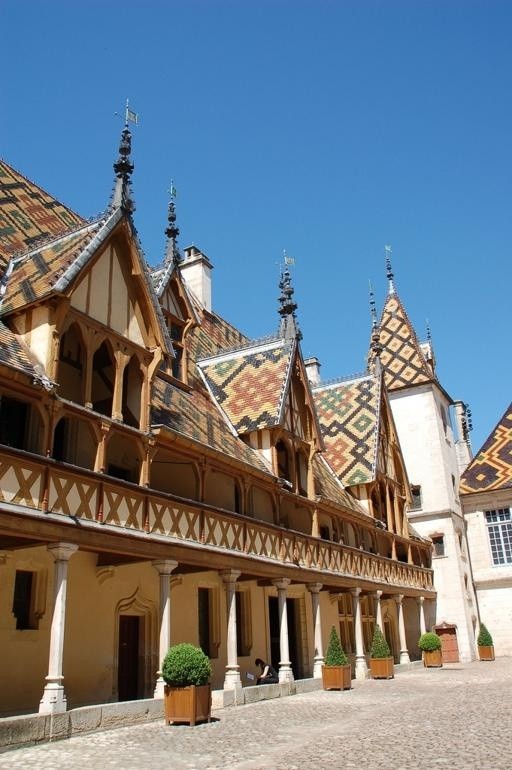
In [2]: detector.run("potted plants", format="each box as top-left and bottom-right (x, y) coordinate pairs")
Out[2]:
(419, 633), (441, 668)
(370, 623), (394, 680)
(162, 642), (212, 724)
(478, 622), (495, 661)
(322, 625), (352, 692)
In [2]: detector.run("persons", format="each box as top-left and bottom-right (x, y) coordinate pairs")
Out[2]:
(255, 658), (279, 685)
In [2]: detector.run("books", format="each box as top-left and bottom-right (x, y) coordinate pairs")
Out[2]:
(246, 672), (255, 681)
